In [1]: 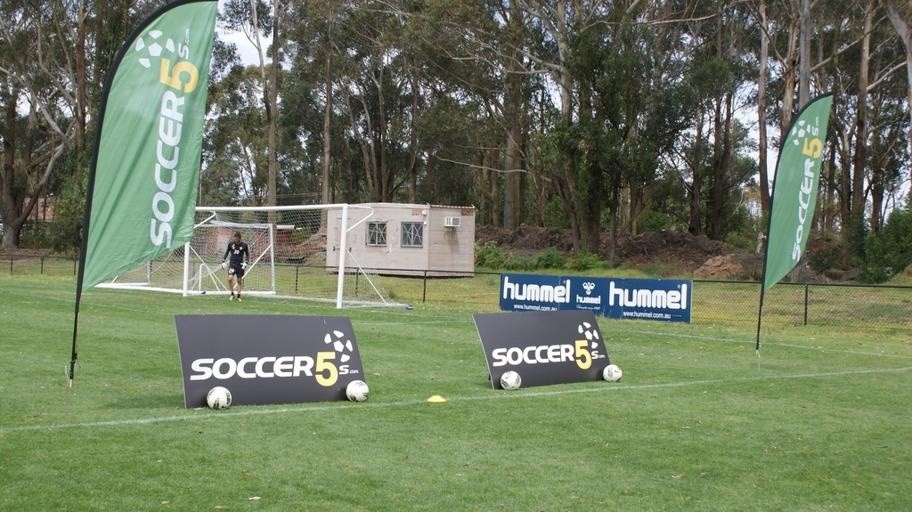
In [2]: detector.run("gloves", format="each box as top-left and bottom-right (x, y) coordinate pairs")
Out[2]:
(221, 261), (227, 270)
(240, 261), (248, 270)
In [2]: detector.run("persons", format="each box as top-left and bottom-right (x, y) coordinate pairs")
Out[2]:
(221, 231), (250, 302)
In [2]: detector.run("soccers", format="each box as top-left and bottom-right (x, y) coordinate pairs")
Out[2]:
(206, 386), (232, 410)
(345, 379), (370, 402)
(603, 365), (623, 383)
(501, 370), (522, 390)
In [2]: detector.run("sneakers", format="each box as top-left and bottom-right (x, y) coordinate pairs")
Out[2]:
(237, 299), (242, 302)
(229, 295), (234, 300)
